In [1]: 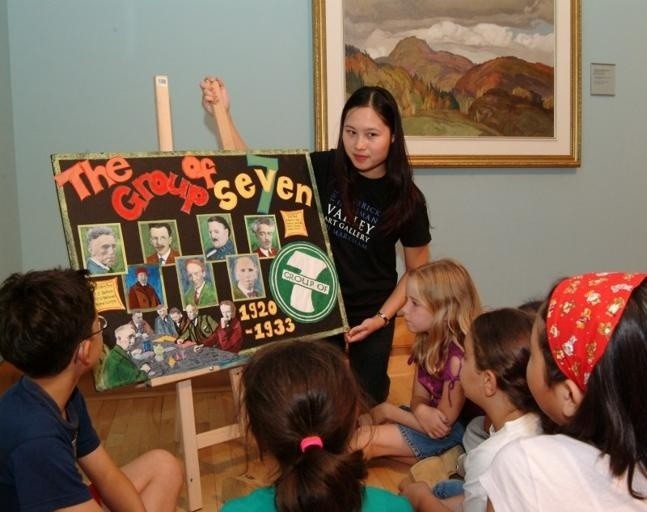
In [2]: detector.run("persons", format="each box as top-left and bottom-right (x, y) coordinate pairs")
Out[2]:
(85, 227), (118, 274)
(128, 267), (161, 309)
(478, 271), (647, 512)
(102, 324), (150, 389)
(434, 299), (544, 499)
(199, 74), (432, 412)
(128, 311), (153, 336)
(153, 300), (244, 354)
(217, 338), (414, 512)
(204, 215), (236, 262)
(146, 222), (180, 264)
(231, 257), (264, 300)
(250, 217), (278, 258)
(184, 257), (217, 309)
(349, 257), (482, 464)
(397, 307), (554, 512)
(0, 266), (184, 512)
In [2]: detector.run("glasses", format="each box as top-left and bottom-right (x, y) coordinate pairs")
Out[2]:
(75, 315), (108, 363)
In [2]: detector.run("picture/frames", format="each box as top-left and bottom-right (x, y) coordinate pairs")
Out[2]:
(311, 0), (582, 168)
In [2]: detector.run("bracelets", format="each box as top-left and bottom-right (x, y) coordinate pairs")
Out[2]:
(376, 311), (391, 327)
